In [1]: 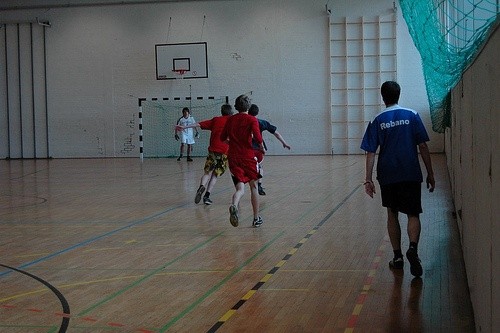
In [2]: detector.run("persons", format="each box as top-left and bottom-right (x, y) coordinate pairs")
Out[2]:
(174, 107), (200, 162)
(360, 80), (436, 277)
(225, 94), (264, 227)
(174, 105), (236, 205)
(249, 104), (291, 196)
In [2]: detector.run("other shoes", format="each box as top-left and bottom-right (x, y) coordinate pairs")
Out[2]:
(177, 157), (182, 161)
(187, 159), (193, 162)
(195, 186), (205, 204)
(389, 257), (404, 269)
(406, 249), (423, 275)
(258, 185), (265, 195)
(203, 197), (212, 205)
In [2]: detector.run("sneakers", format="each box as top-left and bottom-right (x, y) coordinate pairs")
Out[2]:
(229, 205), (239, 227)
(252, 216), (263, 227)
